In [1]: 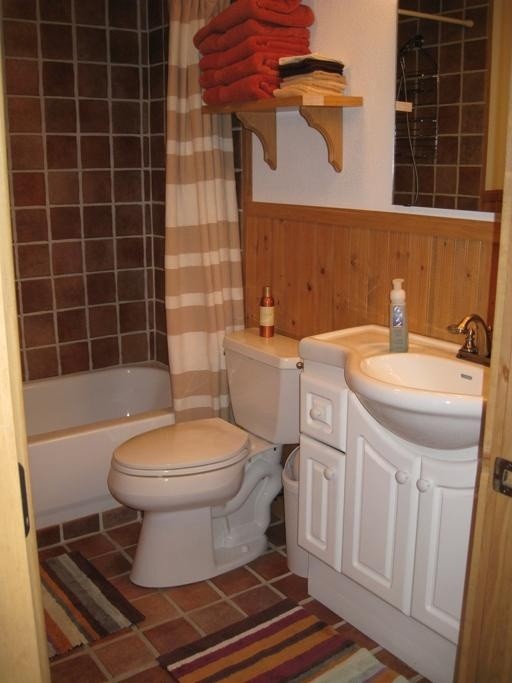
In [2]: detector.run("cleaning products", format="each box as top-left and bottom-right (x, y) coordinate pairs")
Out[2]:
(258, 285), (276, 337)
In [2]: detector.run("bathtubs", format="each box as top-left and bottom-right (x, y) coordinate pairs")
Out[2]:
(24, 360), (174, 531)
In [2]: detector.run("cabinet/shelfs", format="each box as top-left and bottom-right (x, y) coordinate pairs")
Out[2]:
(296, 372), (348, 575)
(342, 391), (478, 683)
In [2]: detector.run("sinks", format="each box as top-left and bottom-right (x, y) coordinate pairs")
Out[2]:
(345, 346), (489, 449)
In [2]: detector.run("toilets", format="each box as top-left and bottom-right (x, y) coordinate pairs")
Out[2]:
(105, 326), (300, 588)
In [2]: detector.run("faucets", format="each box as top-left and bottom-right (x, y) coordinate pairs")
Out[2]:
(447, 313), (492, 360)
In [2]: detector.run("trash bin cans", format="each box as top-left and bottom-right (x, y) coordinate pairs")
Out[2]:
(280, 446), (307, 579)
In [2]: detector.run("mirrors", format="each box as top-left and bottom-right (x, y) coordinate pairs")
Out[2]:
(390, 0), (510, 213)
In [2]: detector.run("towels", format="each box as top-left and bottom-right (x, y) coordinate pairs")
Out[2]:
(191, 0), (349, 105)
